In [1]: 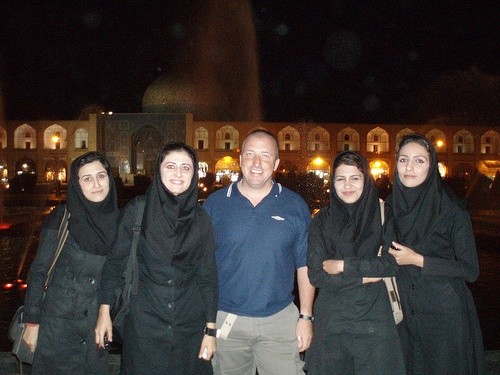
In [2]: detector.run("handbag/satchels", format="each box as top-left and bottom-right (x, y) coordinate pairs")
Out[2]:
(104, 256), (138, 354)
(378, 197), (403, 325)
(9, 307), (36, 364)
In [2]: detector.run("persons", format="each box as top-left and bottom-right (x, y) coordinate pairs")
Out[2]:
(21, 129), (481, 375)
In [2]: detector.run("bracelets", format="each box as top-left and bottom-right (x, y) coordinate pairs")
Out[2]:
(299, 314), (315, 322)
(204, 327), (216, 337)
